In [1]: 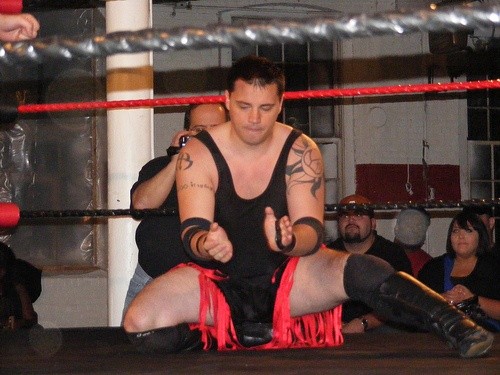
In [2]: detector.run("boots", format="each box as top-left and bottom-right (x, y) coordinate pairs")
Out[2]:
(176, 321), (272, 356)
(369, 272), (494, 358)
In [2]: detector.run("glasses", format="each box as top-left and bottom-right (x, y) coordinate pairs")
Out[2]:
(338, 212), (369, 221)
(189, 126), (204, 132)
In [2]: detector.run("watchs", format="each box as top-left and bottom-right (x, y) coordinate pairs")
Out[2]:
(356, 315), (369, 331)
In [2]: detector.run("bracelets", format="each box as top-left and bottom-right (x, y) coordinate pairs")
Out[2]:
(180, 216), (214, 262)
(275, 215), (324, 257)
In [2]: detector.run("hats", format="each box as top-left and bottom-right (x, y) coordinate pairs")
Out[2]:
(340, 195), (373, 205)
(394, 209), (427, 246)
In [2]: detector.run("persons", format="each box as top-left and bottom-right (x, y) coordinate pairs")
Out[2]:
(121, 55), (500, 359)
(0, 0), (41, 331)
(392, 207), (436, 284)
(326, 194), (412, 333)
(471, 203), (496, 259)
(414, 198), (499, 331)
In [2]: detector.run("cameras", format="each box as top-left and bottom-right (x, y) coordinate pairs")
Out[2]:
(180, 136), (191, 147)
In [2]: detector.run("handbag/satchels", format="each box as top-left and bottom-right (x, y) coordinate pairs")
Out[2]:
(454, 296), (489, 323)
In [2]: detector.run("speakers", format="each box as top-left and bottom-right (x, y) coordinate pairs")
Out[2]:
(429, 0), (467, 53)
